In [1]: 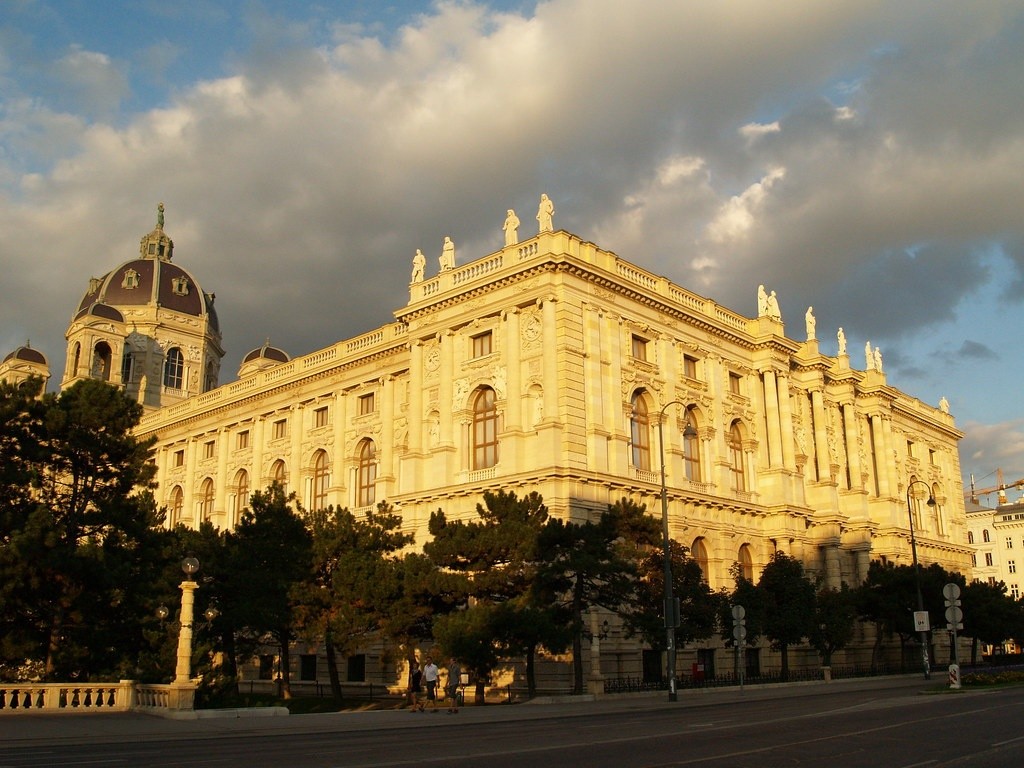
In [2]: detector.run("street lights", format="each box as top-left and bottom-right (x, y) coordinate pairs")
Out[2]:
(156, 557), (217, 685)
(906, 480), (936, 680)
(658, 401), (698, 702)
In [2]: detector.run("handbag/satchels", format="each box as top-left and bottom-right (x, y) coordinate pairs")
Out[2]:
(411, 687), (414, 693)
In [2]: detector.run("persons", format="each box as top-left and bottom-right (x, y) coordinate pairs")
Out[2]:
(805, 306), (816, 340)
(445, 658), (462, 714)
(412, 249), (426, 281)
(503, 210), (520, 245)
(864, 342), (875, 369)
(406, 662), (425, 712)
(537, 193), (554, 231)
(757, 284), (767, 317)
(442, 237), (455, 269)
(419, 656), (440, 712)
(939, 398), (949, 413)
(837, 327), (847, 355)
(873, 347), (882, 370)
(767, 290), (781, 321)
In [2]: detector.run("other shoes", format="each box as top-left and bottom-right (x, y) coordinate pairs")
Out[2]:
(419, 707), (424, 712)
(447, 710), (452, 714)
(410, 709), (416, 713)
(430, 709), (438, 713)
(453, 709), (458, 713)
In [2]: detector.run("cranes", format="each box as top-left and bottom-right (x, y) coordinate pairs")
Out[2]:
(963, 467), (1024, 505)
